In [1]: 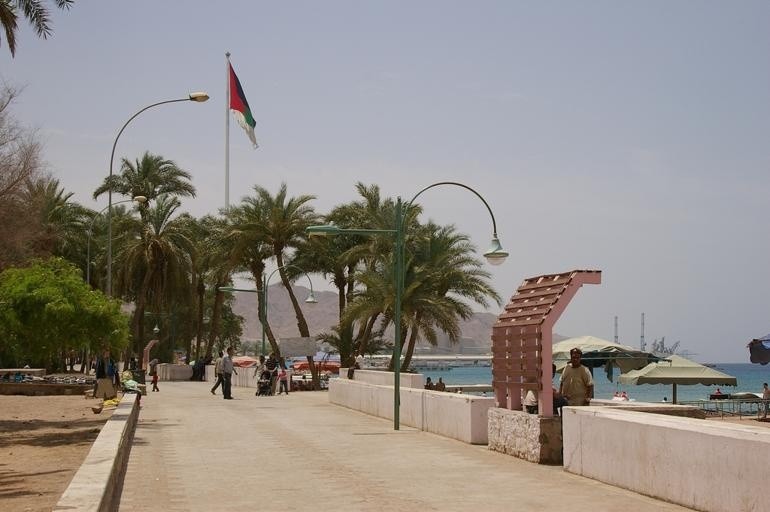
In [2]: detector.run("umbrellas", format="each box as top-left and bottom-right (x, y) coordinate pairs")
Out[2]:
(618, 353), (737, 404)
(550, 335), (672, 398)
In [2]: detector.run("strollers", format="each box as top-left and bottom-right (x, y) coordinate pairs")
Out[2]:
(256, 369), (274, 397)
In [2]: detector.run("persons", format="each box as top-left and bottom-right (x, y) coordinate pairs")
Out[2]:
(436, 376), (445, 391)
(558, 347), (594, 406)
(252, 351), (288, 395)
(151, 371), (159, 392)
(210, 351), (224, 393)
(191, 355), (213, 380)
(614, 392), (629, 400)
(715, 389), (721, 395)
(525, 363), (567, 416)
(222, 347), (238, 399)
(761, 382), (770, 419)
(425, 376), (433, 390)
(130, 356), (136, 370)
(662, 397), (667, 402)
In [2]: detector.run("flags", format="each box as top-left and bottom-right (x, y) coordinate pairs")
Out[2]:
(230, 63), (257, 149)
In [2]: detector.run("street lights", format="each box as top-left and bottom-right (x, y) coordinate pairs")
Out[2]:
(106, 93), (209, 378)
(219, 265), (316, 362)
(304, 182), (507, 431)
(84, 196), (148, 377)
